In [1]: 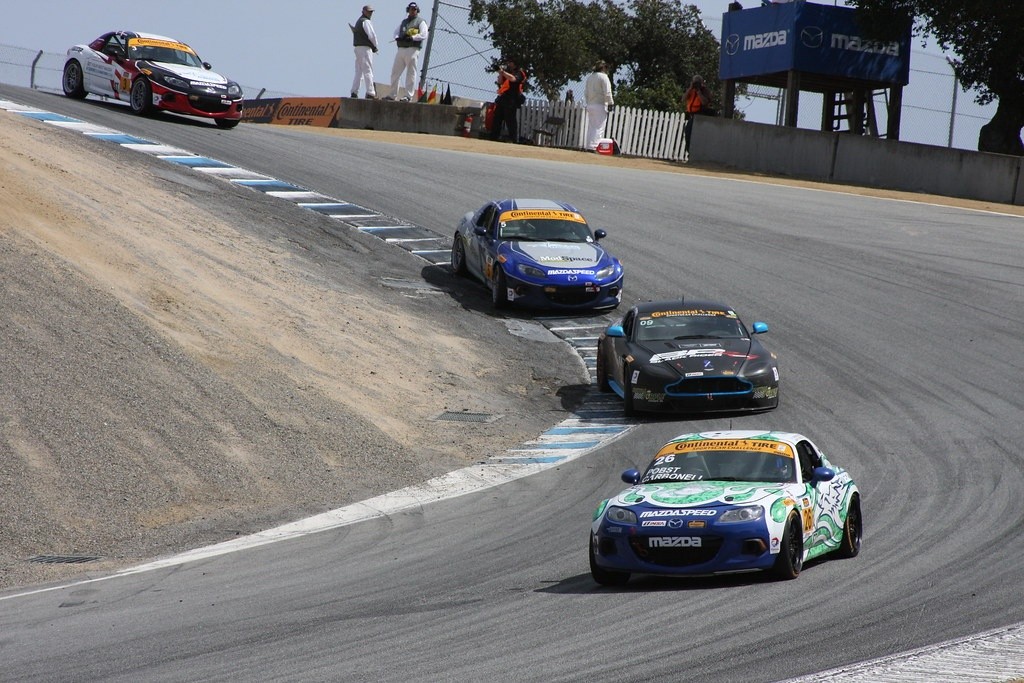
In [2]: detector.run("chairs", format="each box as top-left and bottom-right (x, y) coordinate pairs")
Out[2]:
(529, 117), (566, 147)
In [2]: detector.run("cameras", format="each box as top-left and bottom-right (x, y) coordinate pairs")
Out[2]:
(692, 82), (701, 89)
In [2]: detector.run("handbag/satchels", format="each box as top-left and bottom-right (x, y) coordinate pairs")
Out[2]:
(698, 104), (716, 116)
(518, 92), (525, 104)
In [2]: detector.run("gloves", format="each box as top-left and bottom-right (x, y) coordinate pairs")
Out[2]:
(492, 64), (500, 72)
(608, 105), (613, 111)
(396, 38), (403, 42)
(404, 36), (413, 43)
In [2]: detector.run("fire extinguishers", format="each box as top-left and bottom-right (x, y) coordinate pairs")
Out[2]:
(462, 113), (474, 137)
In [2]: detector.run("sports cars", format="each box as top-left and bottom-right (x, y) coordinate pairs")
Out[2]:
(588, 428), (864, 586)
(449, 198), (625, 312)
(595, 299), (780, 420)
(59, 30), (245, 127)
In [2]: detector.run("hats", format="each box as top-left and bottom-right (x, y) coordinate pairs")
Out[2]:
(409, 3), (417, 8)
(592, 60), (610, 70)
(363, 5), (375, 11)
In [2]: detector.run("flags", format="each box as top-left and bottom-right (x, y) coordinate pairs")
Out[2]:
(418, 81), (452, 105)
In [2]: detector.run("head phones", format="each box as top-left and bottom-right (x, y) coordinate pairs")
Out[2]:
(406, 2), (420, 13)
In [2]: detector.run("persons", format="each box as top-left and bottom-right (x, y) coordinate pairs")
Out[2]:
(682, 75), (711, 164)
(485, 56), (526, 143)
(584, 60), (614, 153)
(382, 2), (428, 102)
(350, 6), (379, 99)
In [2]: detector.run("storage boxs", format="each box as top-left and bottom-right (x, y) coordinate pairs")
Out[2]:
(596, 138), (613, 155)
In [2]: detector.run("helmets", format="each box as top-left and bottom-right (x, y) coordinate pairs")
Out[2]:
(762, 455), (788, 478)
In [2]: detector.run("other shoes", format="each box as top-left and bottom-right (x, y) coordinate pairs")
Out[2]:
(365, 94), (379, 100)
(400, 97), (410, 102)
(351, 92), (359, 98)
(382, 96), (394, 100)
